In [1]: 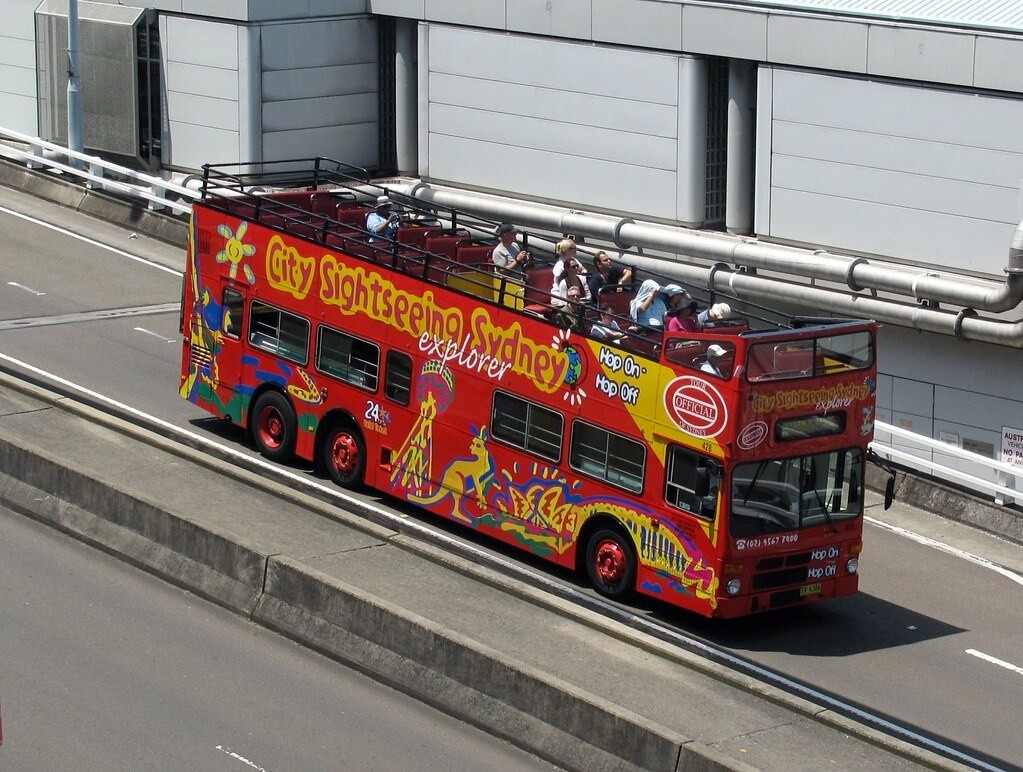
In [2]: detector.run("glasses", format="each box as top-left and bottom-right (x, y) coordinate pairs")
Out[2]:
(570, 264), (579, 269)
(381, 204), (392, 209)
(568, 294), (581, 298)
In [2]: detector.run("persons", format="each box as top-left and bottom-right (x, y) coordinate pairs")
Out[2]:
(630, 280), (668, 331)
(550, 239), (588, 305)
(557, 259), (592, 308)
(696, 303), (731, 331)
(492, 223), (531, 286)
(698, 344), (727, 379)
(590, 303), (638, 347)
(663, 284), (692, 313)
(590, 250), (632, 304)
(556, 285), (591, 335)
(668, 297), (704, 350)
(367, 196), (401, 243)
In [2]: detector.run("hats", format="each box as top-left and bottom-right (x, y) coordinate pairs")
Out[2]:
(500, 224), (519, 234)
(667, 294), (698, 316)
(712, 303), (731, 319)
(375, 196), (394, 209)
(707, 344), (727, 357)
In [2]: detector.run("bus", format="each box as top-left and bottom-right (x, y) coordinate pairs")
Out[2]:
(173, 154), (897, 632)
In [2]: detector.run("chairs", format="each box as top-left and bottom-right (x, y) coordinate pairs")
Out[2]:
(203, 191), (825, 378)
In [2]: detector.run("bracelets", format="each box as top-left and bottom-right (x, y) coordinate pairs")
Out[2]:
(515, 257), (520, 262)
